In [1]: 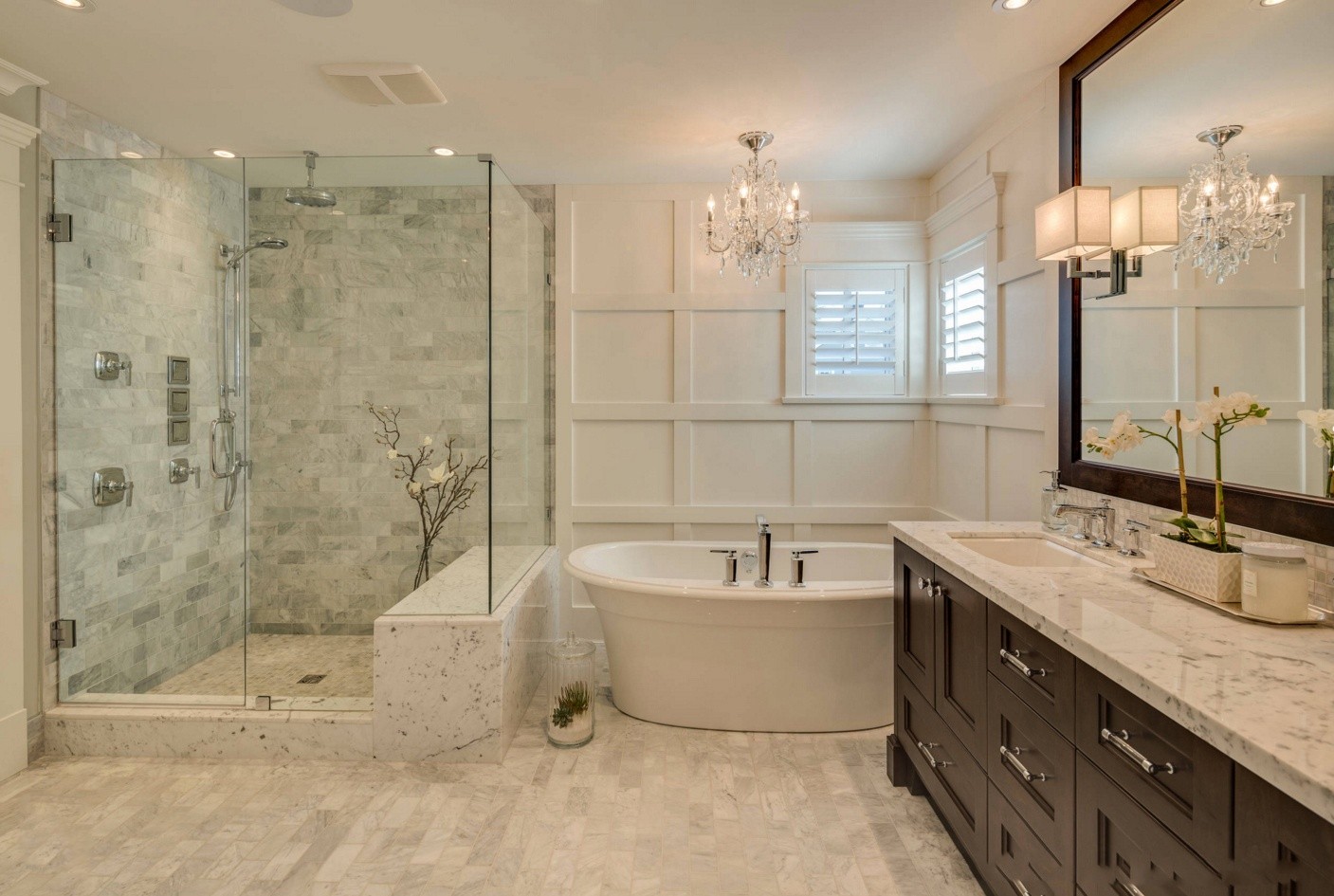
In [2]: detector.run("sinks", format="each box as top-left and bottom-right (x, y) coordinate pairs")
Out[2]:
(947, 528), (1126, 571)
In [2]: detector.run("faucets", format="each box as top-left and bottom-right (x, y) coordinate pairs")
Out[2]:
(1052, 503), (1119, 551)
(754, 514), (774, 588)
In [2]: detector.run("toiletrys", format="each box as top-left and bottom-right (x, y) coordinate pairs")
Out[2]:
(1038, 469), (1069, 532)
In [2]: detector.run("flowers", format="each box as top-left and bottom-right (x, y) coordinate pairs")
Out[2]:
(365, 399), (488, 594)
(1297, 408), (1334, 500)
(1079, 385), (1272, 552)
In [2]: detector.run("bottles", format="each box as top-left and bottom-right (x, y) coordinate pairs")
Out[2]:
(1241, 541), (1309, 622)
(545, 630), (596, 749)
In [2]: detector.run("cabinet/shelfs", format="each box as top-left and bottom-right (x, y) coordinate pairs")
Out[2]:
(887, 538), (1334, 896)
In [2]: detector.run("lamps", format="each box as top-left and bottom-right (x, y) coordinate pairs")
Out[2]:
(698, 127), (810, 287)
(1034, 185), (1118, 301)
(1163, 123), (1296, 285)
(1095, 184), (1179, 301)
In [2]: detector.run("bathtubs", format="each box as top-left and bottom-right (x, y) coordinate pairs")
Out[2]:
(565, 538), (894, 734)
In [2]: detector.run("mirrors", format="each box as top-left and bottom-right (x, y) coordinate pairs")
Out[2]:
(1056, 0), (1334, 548)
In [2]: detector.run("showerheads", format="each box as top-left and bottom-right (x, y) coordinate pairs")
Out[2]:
(227, 237), (289, 267)
(283, 189), (337, 207)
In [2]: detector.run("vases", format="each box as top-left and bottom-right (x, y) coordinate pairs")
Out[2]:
(1148, 533), (1242, 603)
